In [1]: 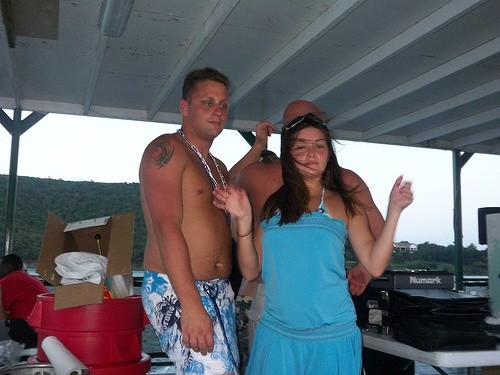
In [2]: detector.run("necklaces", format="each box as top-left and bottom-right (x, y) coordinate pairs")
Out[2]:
(176, 128), (229, 218)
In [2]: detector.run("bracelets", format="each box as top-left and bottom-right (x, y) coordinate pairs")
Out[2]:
(235, 226), (254, 239)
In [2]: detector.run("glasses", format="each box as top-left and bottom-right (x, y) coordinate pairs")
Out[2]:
(285, 114), (326, 141)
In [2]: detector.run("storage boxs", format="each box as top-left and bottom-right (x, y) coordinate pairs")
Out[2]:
(36, 211), (136, 311)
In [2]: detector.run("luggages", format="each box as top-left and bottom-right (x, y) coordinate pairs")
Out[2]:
(390, 289), (491, 352)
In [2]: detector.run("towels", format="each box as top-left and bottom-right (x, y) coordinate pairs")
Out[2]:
(54, 252), (108, 285)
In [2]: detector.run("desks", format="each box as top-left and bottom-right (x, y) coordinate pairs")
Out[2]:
(362, 328), (500, 375)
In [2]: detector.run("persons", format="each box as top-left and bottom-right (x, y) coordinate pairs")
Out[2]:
(237, 98), (388, 375)
(0, 253), (50, 362)
(138, 65), (277, 375)
(211, 111), (413, 375)
